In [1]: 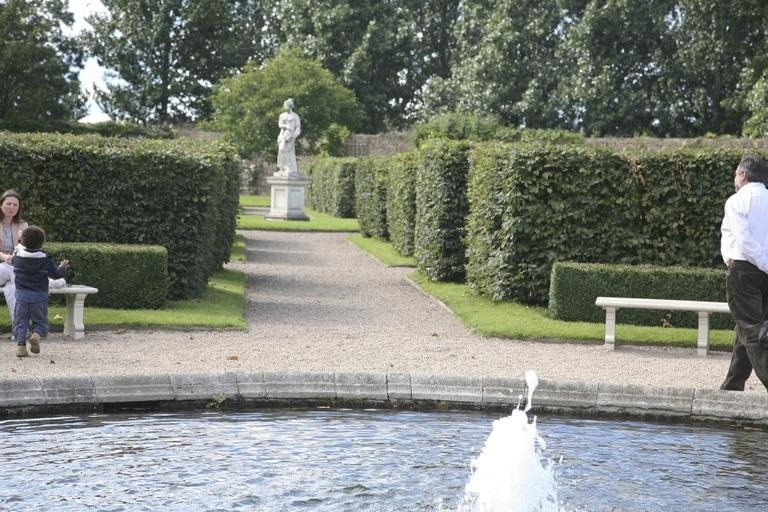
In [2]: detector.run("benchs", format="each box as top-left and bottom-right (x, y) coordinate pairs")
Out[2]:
(593, 295), (734, 357)
(0, 282), (99, 341)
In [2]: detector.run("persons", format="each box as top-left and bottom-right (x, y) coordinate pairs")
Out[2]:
(12, 227), (71, 358)
(721, 154), (767, 391)
(274, 98), (302, 180)
(0, 189), (31, 341)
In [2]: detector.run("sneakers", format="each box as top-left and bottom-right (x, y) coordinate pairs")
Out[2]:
(28, 331), (41, 354)
(14, 343), (29, 357)
(48, 276), (67, 289)
